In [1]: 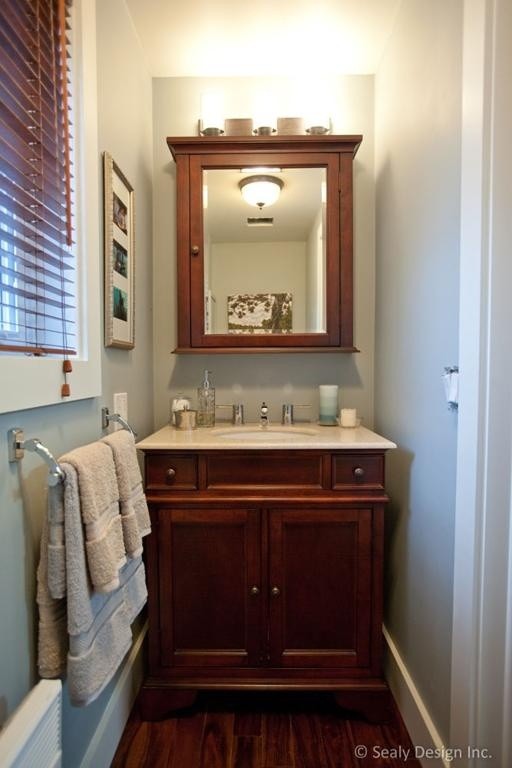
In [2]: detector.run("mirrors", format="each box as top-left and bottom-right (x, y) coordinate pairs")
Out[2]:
(200, 165), (327, 336)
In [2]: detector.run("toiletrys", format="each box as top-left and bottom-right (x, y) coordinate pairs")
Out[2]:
(197, 369), (216, 428)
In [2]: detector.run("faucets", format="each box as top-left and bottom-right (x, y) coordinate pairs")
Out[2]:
(259, 402), (270, 428)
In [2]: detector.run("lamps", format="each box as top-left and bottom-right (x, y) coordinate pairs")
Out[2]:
(238, 177), (285, 226)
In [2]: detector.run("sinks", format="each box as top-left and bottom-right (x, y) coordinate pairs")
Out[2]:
(210, 426), (321, 449)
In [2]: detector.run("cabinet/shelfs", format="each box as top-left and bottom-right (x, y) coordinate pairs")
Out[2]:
(167, 133), (364, 357)
(132, 419), (398, 721)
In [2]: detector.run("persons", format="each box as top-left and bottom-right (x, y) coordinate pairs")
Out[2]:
(117, 205), (126, 229)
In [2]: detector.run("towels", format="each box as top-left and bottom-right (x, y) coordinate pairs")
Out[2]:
(99, 429), (156, 627)
(34, 440), (134, 709)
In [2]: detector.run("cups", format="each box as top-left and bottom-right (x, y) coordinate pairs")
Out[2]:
(318, 384), (339, 424)
(282, 404), (293, 424)
(231, 403), (243, 426)
(341, 408), (359, 428)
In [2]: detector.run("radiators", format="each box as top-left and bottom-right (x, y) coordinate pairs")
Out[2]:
(1, 678), (63, 768)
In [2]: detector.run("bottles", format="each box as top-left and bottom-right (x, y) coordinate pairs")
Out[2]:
(174, 404), (197, 429)
(171, 397), (190, 424)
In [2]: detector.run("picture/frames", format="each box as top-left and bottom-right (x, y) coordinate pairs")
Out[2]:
(103, 150), (137, 350)
